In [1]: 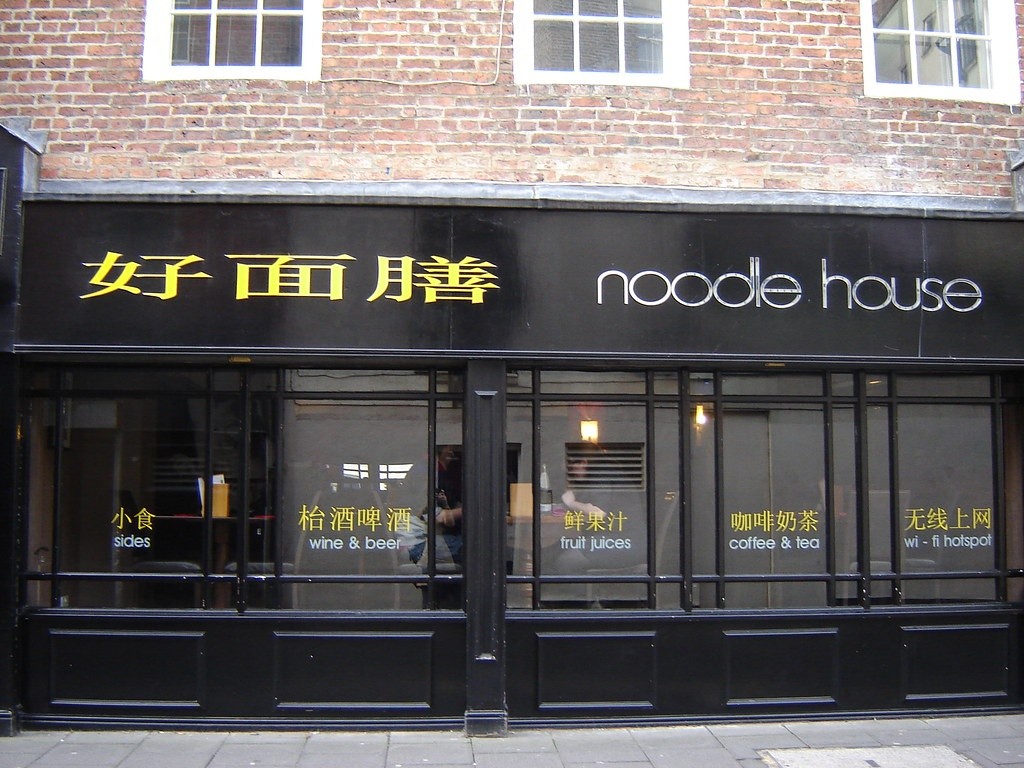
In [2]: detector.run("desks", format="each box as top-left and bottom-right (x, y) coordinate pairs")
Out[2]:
(506, 508), (581, 539)
(146, 516), (273, 611)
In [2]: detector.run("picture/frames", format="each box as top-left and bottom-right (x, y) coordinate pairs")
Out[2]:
(899, 9), (979, 86)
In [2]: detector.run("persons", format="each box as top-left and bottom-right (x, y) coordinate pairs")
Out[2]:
(545, 443), (645, 575)
(397, 445), (462, 576)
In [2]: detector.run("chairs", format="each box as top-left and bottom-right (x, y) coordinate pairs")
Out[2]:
(130, 490), (978, 609)
